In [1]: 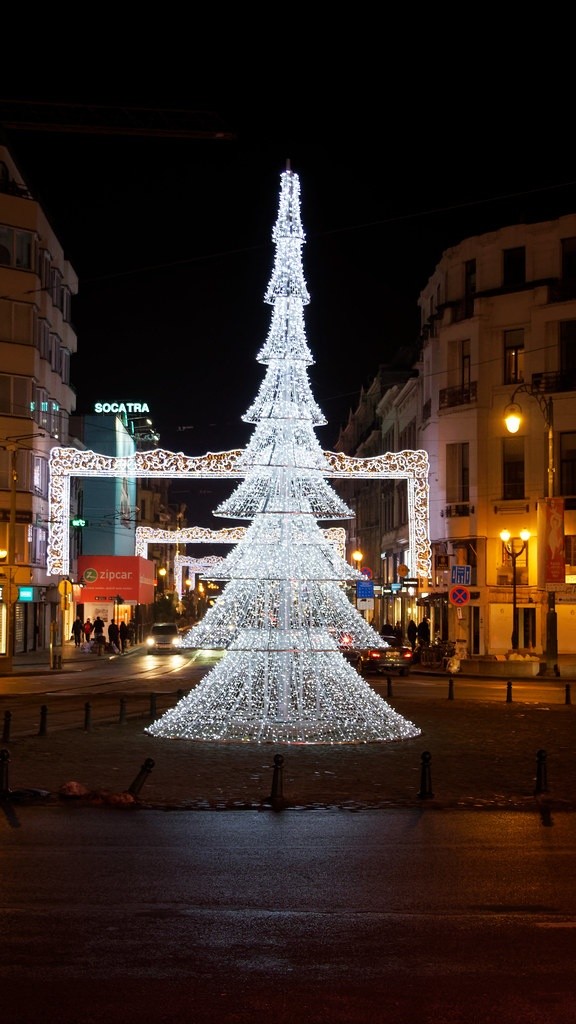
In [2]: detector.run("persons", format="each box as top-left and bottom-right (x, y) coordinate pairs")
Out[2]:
(382, 620), (393, 641)
(120, 621), (127, 653)
(370, 618), (377, 629)
(127, 620), (134, 646)
(93, 617), (104, 642)
(72, 616), (83, 647)
(418, 617), (429, 645)
(108, 619), (121, 654)
(408, 620), (417, 651)
(83, 618), (93, 643)
(394, 621), (405, 645)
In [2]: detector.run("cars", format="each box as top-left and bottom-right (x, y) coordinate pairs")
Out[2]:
(339, 635), (413, 676)
(147, 623), (182, 654)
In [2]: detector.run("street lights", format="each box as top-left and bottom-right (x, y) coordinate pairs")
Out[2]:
(500, 529), (530, 648)
(499, 382), (562, 680)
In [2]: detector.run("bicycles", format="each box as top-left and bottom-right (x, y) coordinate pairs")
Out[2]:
(420, 637), (456, 669)
